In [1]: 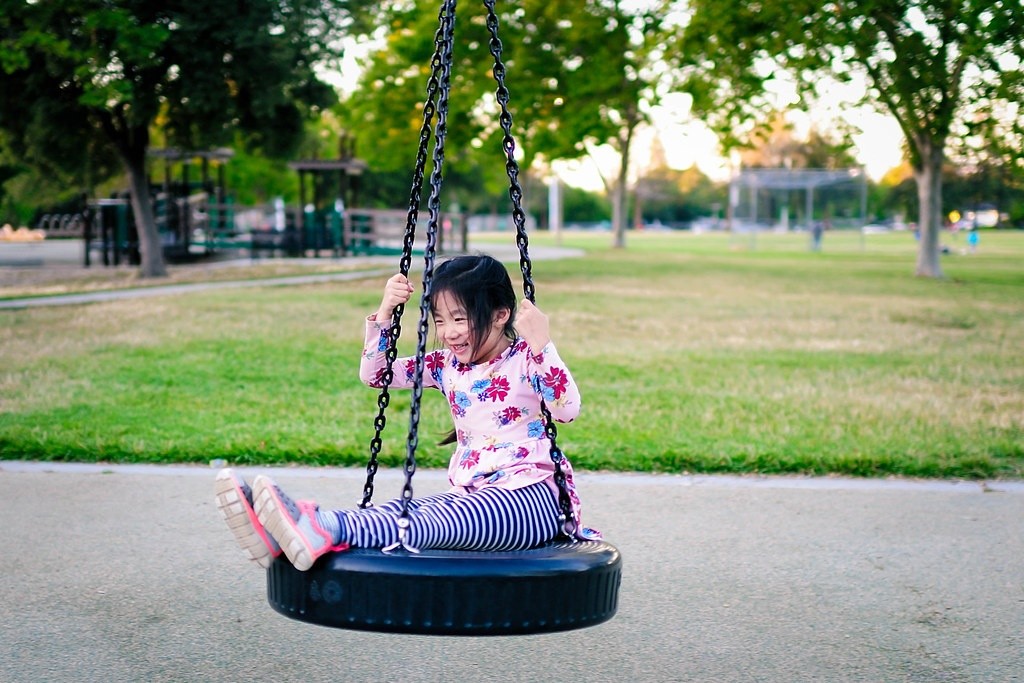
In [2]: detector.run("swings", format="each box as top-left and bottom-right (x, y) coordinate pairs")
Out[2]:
(265, 0), (621, 639)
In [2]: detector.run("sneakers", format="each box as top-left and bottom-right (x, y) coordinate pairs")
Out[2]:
(216, 469), (281, 570)
(253, 472), (334, 569)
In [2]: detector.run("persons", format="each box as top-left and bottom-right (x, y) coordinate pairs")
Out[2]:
(215, 256), (604, 571)
